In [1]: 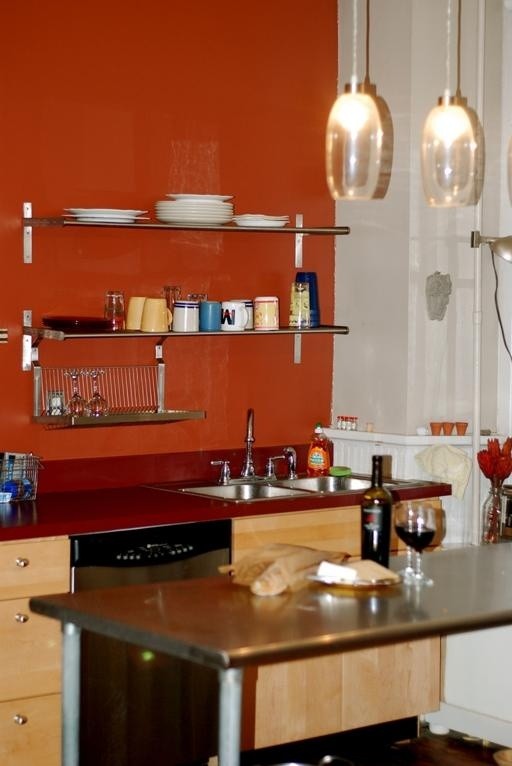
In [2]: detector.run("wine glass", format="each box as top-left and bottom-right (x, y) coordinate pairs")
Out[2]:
(64, 368), (110, 418)
(393, 500), (436, 588)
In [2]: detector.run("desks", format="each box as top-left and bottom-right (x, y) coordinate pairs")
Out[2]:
(27, 542), (511, 764)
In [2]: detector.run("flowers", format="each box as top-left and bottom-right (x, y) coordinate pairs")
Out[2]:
(474, 437), (512, 546)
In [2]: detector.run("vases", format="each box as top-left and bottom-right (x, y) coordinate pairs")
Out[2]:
(479, 487), (508, 547)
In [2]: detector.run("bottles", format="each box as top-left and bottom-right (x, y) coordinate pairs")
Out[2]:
(359, 454), (392, 569)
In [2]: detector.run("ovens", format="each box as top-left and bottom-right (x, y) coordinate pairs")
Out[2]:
(70, 520), (232, 763)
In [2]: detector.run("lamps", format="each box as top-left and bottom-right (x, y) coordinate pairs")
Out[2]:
(325, 0), (488, 210)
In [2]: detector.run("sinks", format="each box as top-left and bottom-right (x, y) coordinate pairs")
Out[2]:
(269, 474), (426, 497)
(142, 477), (313, 506)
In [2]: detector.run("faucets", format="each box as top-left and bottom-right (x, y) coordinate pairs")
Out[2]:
(239, 407), (255, 476)
(282, 446), (297, 477)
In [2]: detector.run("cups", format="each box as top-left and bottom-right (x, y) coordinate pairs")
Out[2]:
(104, 283), (281, 333)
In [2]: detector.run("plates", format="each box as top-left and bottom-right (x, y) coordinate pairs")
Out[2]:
(62, 191), (290, 229)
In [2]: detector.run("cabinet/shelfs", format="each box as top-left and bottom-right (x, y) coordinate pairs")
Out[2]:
(232, 496), (443, 750)
(2, 533), (72, 764)
